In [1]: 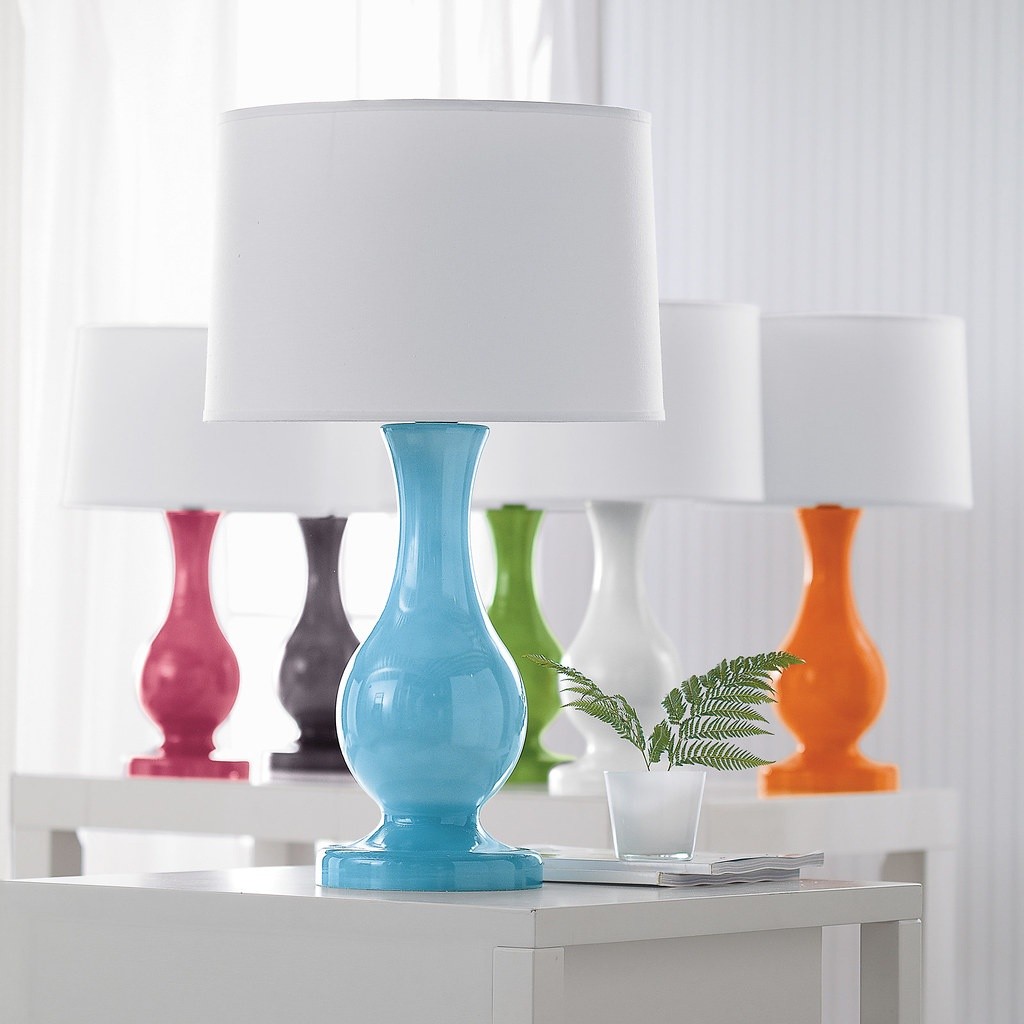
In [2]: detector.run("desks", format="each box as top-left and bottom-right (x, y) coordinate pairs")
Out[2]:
(7, 772), (975, 1024)
(0, 867), (925, 1024)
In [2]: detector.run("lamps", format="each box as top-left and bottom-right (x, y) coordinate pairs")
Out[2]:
(67, 302), (978, 795)
(201, 100), (669, 889)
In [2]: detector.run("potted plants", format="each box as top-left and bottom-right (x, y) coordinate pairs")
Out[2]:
(517, 651), (808, 864)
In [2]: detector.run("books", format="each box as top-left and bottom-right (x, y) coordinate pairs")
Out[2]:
(506, 842), (825, 888)
